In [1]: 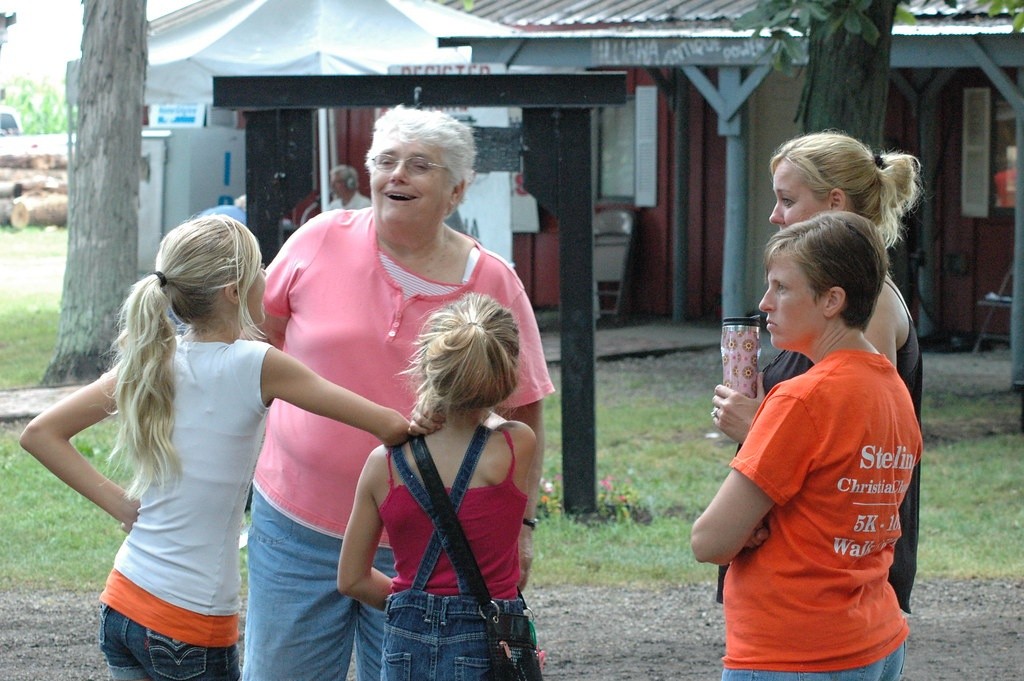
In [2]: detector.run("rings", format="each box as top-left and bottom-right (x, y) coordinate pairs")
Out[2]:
(710, 408), (719, 417)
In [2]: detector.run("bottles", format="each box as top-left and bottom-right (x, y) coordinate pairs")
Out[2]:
(722, 314), (761, 402)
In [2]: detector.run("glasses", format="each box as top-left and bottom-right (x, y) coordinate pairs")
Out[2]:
(372, 154), (447, 176)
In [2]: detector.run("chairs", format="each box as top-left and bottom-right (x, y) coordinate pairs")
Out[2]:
(974, 264), (1012, 356)
(591, 202), (645, 327)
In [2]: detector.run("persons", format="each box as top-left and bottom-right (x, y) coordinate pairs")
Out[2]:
(691, 214), (911, 681)
(199, 195), (248, 225)
(710, 133), (923, 614)
(328, 162), (372, 211)
(336, 292), (539, 681)
(15, 212), (412, 681)
(234, 102), (556, 680)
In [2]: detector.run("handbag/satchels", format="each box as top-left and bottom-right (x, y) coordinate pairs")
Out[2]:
(479, 599), (547, 681)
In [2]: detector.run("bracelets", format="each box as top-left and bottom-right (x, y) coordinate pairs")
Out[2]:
(522, 518), (538, 530)
